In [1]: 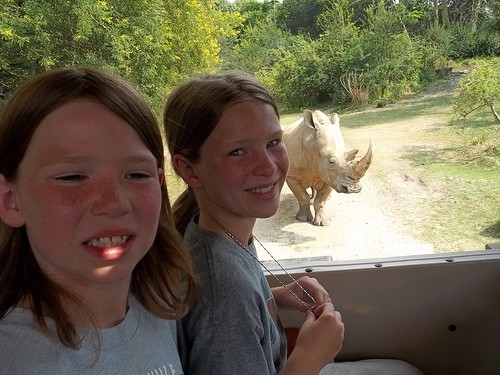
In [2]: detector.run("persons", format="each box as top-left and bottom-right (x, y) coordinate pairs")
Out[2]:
(163, 71), (425, 375)
(1, 66), (199, 375)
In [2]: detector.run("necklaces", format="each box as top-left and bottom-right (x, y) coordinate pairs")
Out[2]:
(201, 211), (317, 311)
